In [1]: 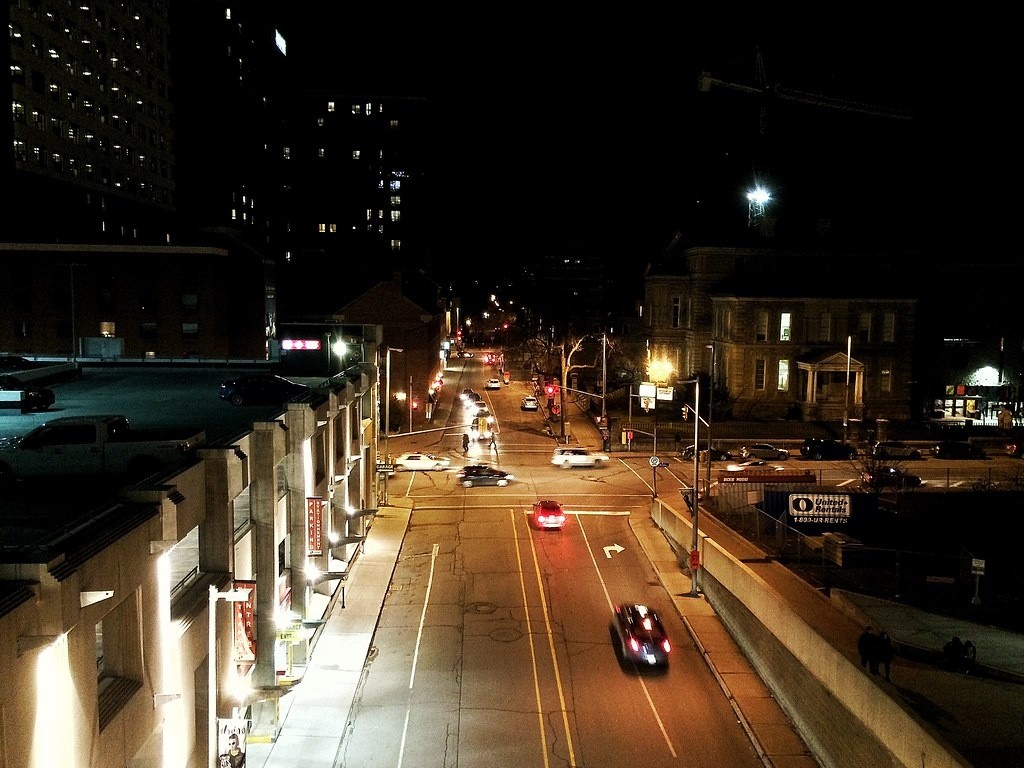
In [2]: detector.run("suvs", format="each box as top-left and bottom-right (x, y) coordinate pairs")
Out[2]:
(800, 436), (858, 461)
(551, 446), (610, 468)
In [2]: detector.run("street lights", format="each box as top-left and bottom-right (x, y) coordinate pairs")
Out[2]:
(647, 358), (700, 596)
(384, 346), (403, 507)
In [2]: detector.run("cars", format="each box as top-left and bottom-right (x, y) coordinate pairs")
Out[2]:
(487, 379), (500, 390)
(456, 351), (473, 358)
(614, 602), (673, 673)
(859, 465), (922, 488)
(726, 458), (786, 473)
(460, 386), (496, 442)
(872, 440), (923, 461)
(532, 499), (567, 531)
(521, 396), (538, 410)
(681, 443), (732, 462)
(930, 439), (986, 460)
(739, 443), (790, 461)
(393, 450), (452, 472)
(456, 465), (515, 488)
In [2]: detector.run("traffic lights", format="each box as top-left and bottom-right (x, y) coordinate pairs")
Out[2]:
(548, 386), (554, 399)
(682, 405), (688, 421)
